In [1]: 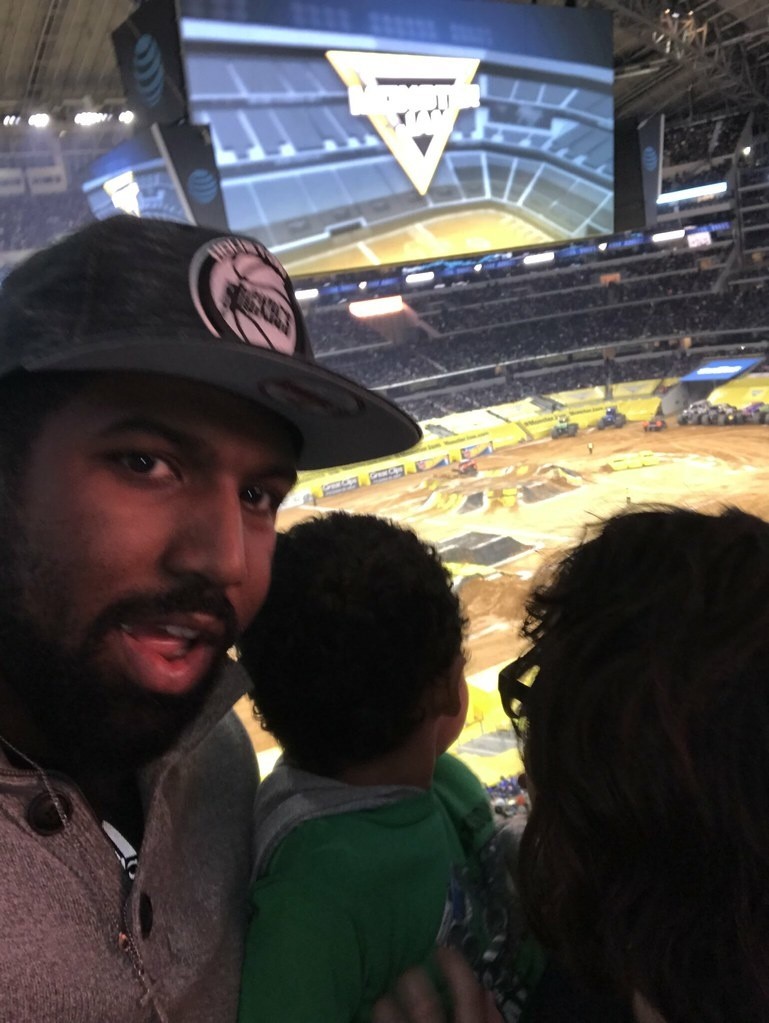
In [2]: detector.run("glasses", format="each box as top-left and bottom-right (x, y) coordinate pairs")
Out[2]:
(499, 645), (541, 720)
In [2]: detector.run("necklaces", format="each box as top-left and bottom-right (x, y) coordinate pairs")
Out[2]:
(0, 739), (168, 1023)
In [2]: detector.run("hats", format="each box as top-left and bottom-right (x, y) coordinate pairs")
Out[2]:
(0, 214), (424, 472)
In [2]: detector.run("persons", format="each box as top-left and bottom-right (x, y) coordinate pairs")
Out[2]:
(497, 505), (769, 1023)
(498, 775), (521, 798)
(237, 512), (517, 1023)
(588, 442), (594, 454)
(0, 191), (98, 284)
(0, 213), (487, 1023)
(289, 107), (769, 427)
